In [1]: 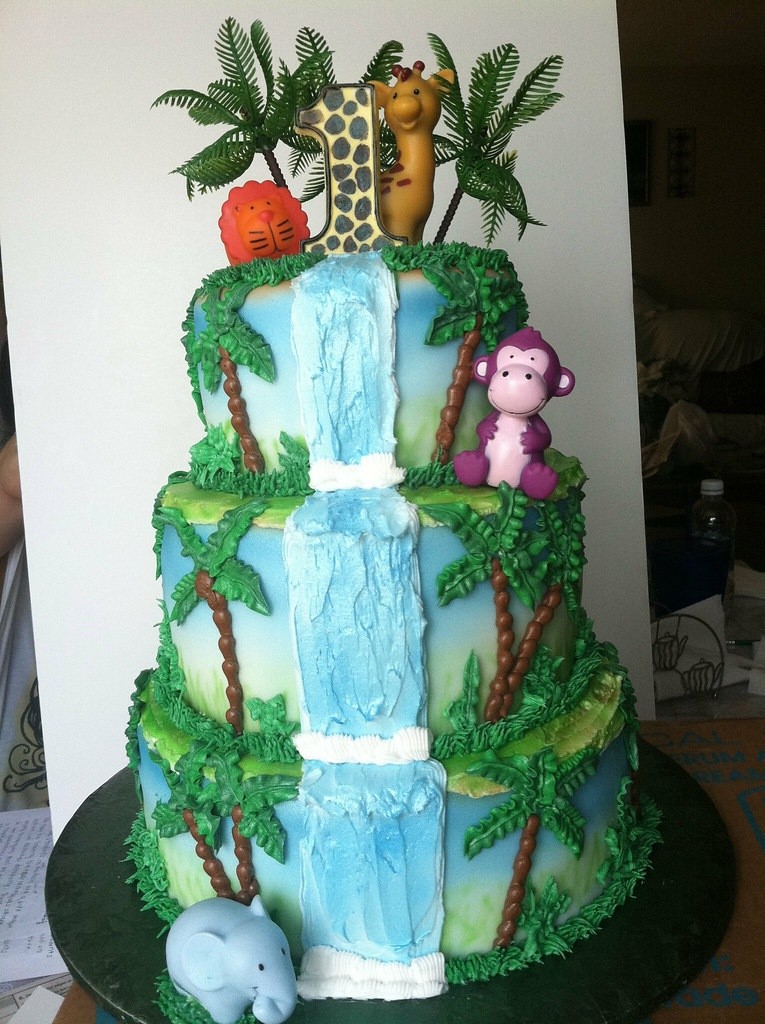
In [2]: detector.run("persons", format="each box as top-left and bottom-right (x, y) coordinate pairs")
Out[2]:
(0, 248), (49, 815)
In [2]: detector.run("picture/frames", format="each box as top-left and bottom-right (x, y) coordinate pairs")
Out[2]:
(624, 119), (652, 207)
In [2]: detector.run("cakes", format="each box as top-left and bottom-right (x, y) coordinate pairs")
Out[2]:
(125, 15), (661, 1000)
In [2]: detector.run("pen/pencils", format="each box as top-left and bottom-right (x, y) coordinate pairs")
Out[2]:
(725, 640), (760, 645)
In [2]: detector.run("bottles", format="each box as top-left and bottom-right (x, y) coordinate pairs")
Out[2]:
(690, 479), (736, 588)
